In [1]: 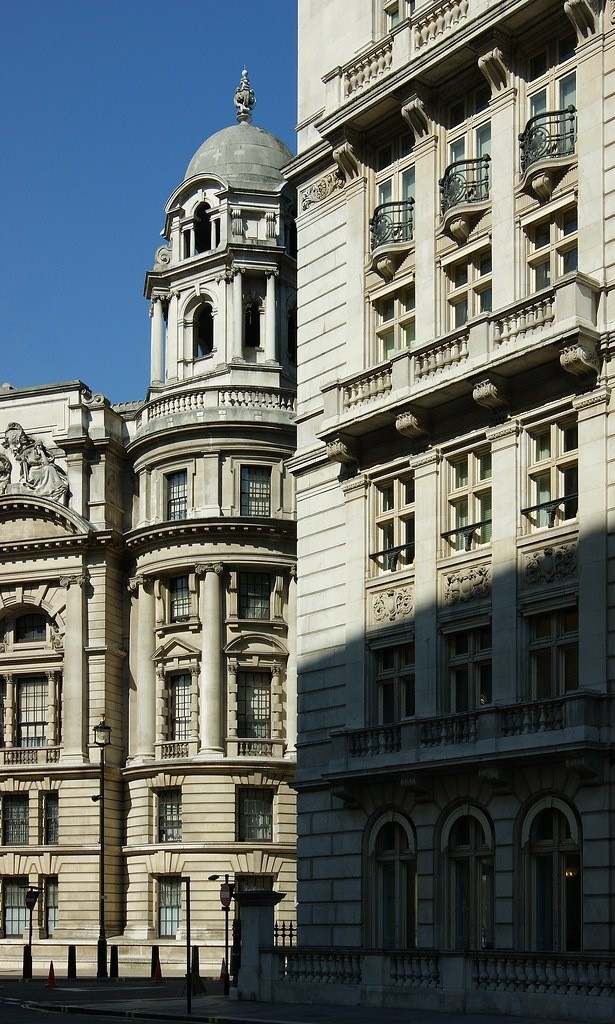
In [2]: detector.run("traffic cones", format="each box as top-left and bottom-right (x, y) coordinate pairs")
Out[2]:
(217, 958), (226, 983)
(45, 960), (60, 987)
(151, 958), (166, 984)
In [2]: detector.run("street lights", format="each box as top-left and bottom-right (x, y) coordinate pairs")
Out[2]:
(92, 720), (113, 980)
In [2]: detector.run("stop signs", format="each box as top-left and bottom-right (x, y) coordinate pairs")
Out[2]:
(26, 890), (36, 910)
(220, 883), (231, 907)
